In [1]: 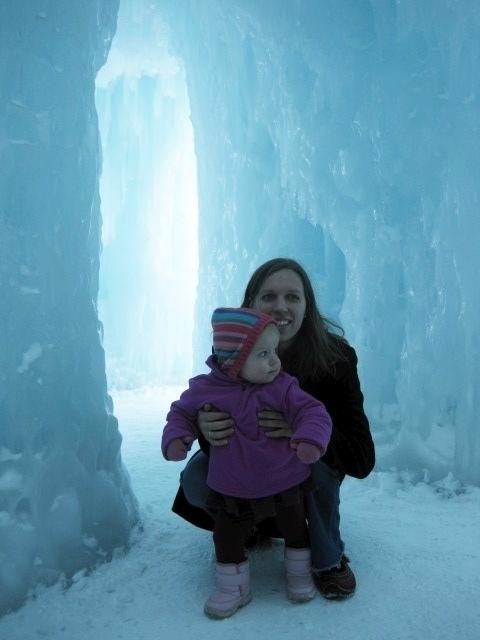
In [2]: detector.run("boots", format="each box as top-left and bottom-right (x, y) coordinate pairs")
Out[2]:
(281, 546), (319, 603)
(202, 559), (255, 620)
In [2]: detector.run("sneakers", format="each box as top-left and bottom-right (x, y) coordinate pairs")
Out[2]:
(310, 552), (357, 601)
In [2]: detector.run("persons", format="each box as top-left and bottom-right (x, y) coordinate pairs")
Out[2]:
(169, 258), (378, 601)
(160, 305), (336, 620)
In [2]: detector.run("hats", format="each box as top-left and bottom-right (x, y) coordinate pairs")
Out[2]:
(211, 306), (279, 381)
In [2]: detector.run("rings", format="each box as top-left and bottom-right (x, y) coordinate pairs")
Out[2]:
(206, 430), (213, 439)
